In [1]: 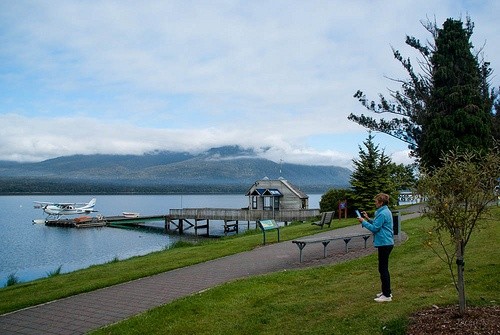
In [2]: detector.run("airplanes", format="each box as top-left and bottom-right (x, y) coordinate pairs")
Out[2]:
(33, 198), (100, 218)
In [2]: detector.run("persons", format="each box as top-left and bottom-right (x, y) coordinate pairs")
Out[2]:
(357, 193), (395, 302)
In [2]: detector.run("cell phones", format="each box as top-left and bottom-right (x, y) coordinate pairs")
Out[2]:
(356, 210), (361, 217)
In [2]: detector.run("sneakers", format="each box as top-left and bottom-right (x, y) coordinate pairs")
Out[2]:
(374, 292), (393, 302)
